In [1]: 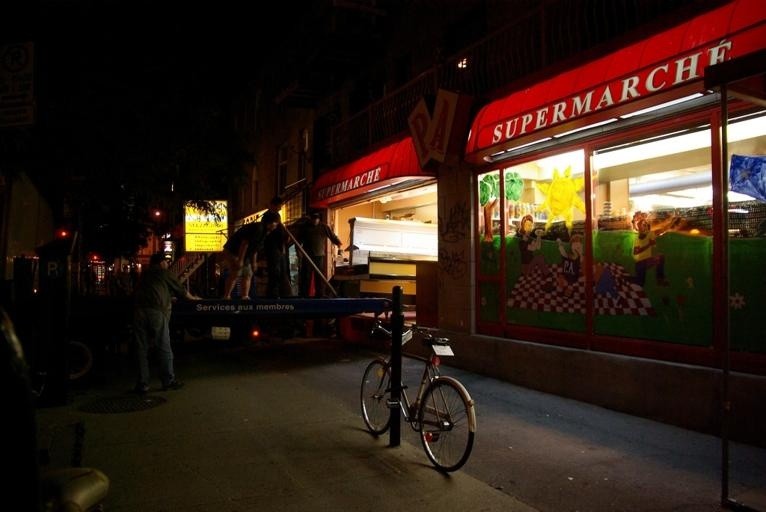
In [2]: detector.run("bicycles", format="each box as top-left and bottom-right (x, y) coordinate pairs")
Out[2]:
(359, 319), (476, 472)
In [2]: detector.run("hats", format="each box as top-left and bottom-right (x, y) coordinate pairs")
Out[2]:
(311, 212), (320, 218)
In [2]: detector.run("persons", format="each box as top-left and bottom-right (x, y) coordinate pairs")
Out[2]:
(130, 252), (203, 394)
(217, 195), (341, 300)
(0, 305), (110, 512)
(82, 263), (139, 297)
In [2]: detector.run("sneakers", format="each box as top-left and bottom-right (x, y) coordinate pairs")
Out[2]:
(136, 384), (150, 395)
(164, 377), (183, 392)
(223, 295), (249, 301)
